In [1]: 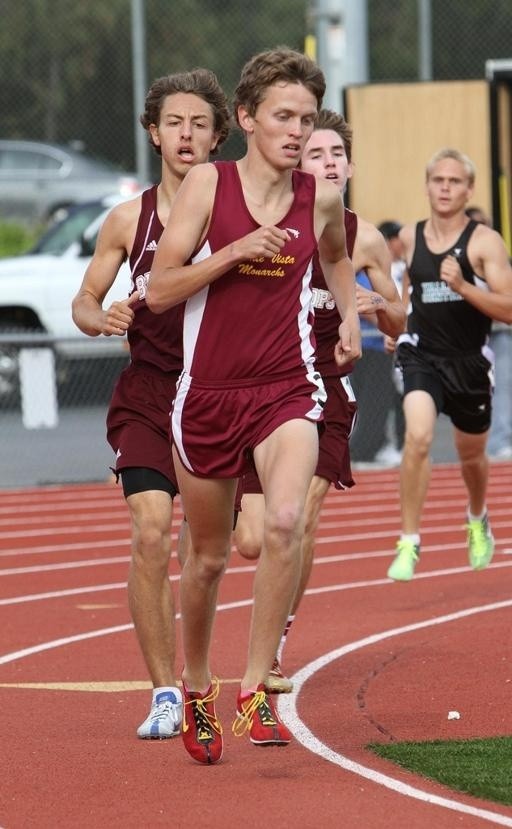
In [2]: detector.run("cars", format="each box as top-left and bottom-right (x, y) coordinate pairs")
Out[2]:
(0, 140), (138, 409)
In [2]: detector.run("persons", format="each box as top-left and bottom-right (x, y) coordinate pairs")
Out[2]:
(68, 64), (237, 744)
(334, 260), (399, 470)
(464, 207), (491, 227)
(380, 146), (511, 584)
(368, 221), (413, 461)
(225, 103), (410, 694)
(140, 44), (366, 768)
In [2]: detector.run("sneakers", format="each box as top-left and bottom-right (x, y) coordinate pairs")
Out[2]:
(264, 653), (295, 694)
(235, 683), (292, 745)
(136, 685), (183, 740)
(180, 664), (225, 767)
(464, 508), (498, 575)
(384, 537), (422, 584)
